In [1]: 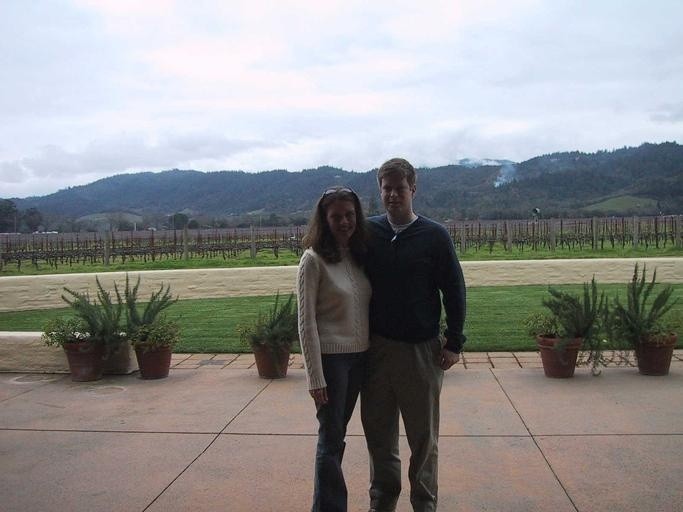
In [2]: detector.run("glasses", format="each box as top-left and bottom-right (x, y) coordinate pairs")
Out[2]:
(322, 185), (355, 196)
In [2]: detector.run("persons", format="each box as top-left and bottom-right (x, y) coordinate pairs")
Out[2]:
(296, 187), (375, 512)
(349, 158), (467, 512)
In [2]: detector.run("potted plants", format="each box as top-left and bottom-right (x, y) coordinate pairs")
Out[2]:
(40, 273), (185, 382)
(240, 288), (298, 379)
(520, 262), (682, 379)
